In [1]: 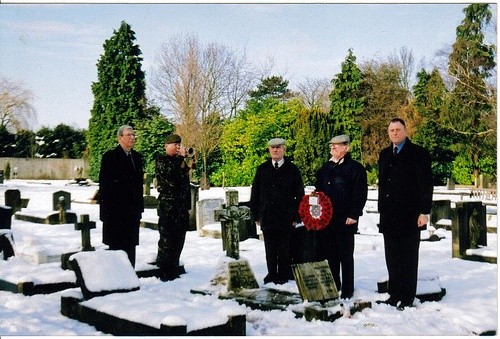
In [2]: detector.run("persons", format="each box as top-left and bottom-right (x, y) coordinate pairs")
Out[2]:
(4, 160), (11, 181)
(250, 137), (304, 285)
(153, 134), (191, 281)
(377, 118), (433, 311)
(309, 134), (367, 300)
(98, 125), (143, 269)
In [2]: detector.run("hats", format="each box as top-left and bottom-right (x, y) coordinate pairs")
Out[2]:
(328, 134), (350, 145)
(268, 138), (286, 147)
(164, 134), (181, 143)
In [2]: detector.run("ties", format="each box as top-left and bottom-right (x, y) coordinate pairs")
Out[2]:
(274, 162), (279, 169)
(128, 153), (132, 160)
(394, 147), (399, 158)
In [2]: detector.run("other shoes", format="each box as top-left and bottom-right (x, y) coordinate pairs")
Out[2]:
(375, 297), (398, 306)
(397, 303), (412, 312)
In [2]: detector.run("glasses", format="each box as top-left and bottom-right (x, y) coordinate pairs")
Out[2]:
(271, 146), (280, 149)
(122, 134), (134, 136)
